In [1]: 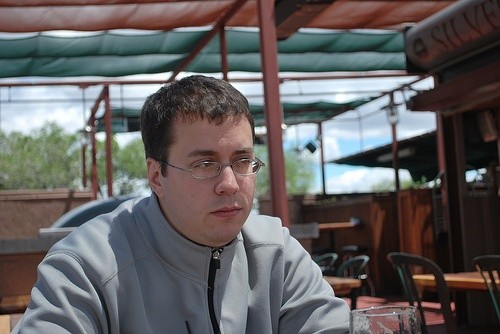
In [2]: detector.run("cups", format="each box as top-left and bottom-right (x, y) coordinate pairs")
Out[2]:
(349, 305), (423, 334)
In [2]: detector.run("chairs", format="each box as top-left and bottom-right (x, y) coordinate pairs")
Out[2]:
(387, 252), (500, 334)
(313, 253), (376, 296)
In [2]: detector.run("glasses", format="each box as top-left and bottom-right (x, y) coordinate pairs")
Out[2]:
(160, 156), (265, 179)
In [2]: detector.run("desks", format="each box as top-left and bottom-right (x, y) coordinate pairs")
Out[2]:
(316, 221), (357, 253)
(324, 276), (361, 295)
(412, 269), (500, 294)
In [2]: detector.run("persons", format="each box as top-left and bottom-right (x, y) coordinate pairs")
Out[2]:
(8, 74), (393, 334)
